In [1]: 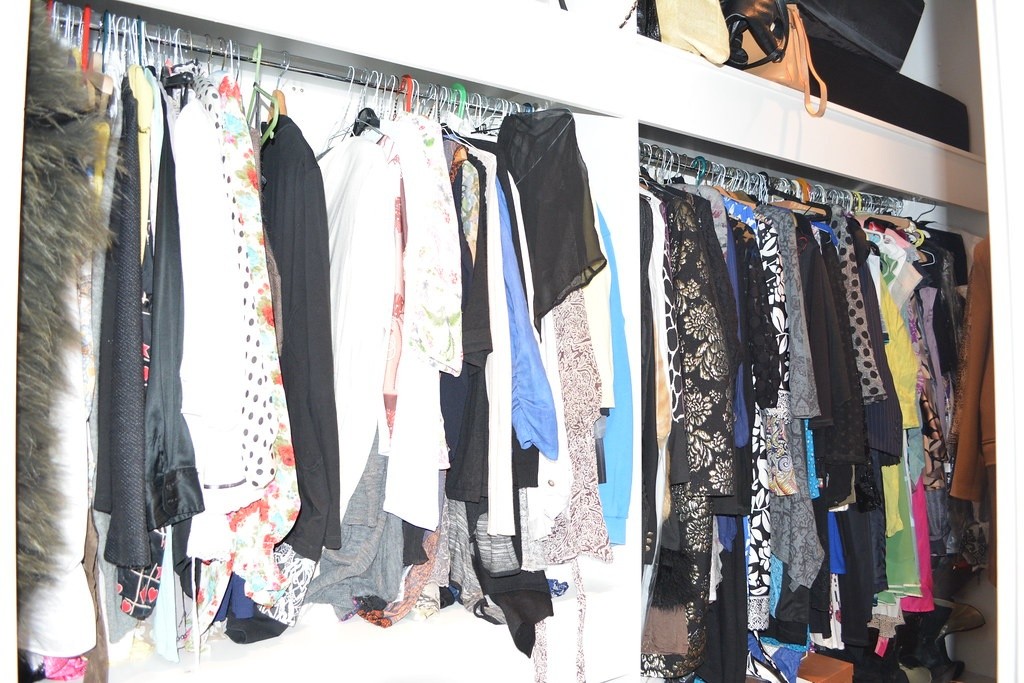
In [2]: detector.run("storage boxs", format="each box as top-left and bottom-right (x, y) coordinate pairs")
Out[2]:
(787, 0), (970, 152)
(797, 652), (855, 683)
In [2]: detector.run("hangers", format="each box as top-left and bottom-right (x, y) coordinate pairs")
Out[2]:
(40, 0), (577, 190)
(640, 137), (937, 265)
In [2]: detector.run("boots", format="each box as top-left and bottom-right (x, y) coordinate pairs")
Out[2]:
(900, 597), (986, 683)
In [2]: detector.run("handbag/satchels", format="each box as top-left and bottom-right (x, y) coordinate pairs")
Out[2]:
(720, 1), (827, 117)
